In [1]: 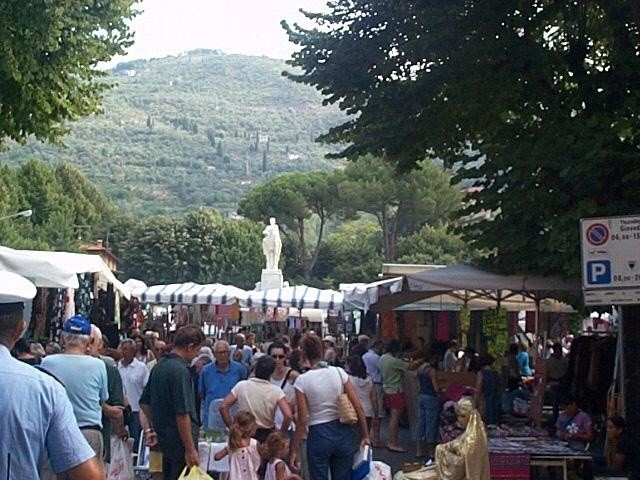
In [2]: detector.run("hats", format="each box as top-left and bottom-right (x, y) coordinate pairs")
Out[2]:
(63, 314), (91, 336)
(358, 335), (369, 343)
(0, 271), (38, 305)
(323, 335), (336, 346)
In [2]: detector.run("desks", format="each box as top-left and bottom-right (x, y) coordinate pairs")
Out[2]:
(438, 426), (597, 479)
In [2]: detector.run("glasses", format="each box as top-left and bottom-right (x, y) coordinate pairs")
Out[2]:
(271, 354), (286, 359)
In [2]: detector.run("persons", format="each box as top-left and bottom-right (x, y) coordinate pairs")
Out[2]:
(12, 317), (640, 478)
(0, 264), (102, 478)
(261, 217), (284, 269)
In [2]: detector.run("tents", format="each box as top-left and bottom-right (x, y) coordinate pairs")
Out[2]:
(0, 245), (625, 416)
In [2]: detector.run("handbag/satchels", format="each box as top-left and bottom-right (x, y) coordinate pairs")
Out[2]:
(336, 366), (358, 425)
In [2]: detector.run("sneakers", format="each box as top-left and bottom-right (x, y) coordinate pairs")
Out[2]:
(370, 440), (436, 459)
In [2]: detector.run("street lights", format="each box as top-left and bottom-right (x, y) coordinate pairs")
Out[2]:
(0, 209), (32, 222)
